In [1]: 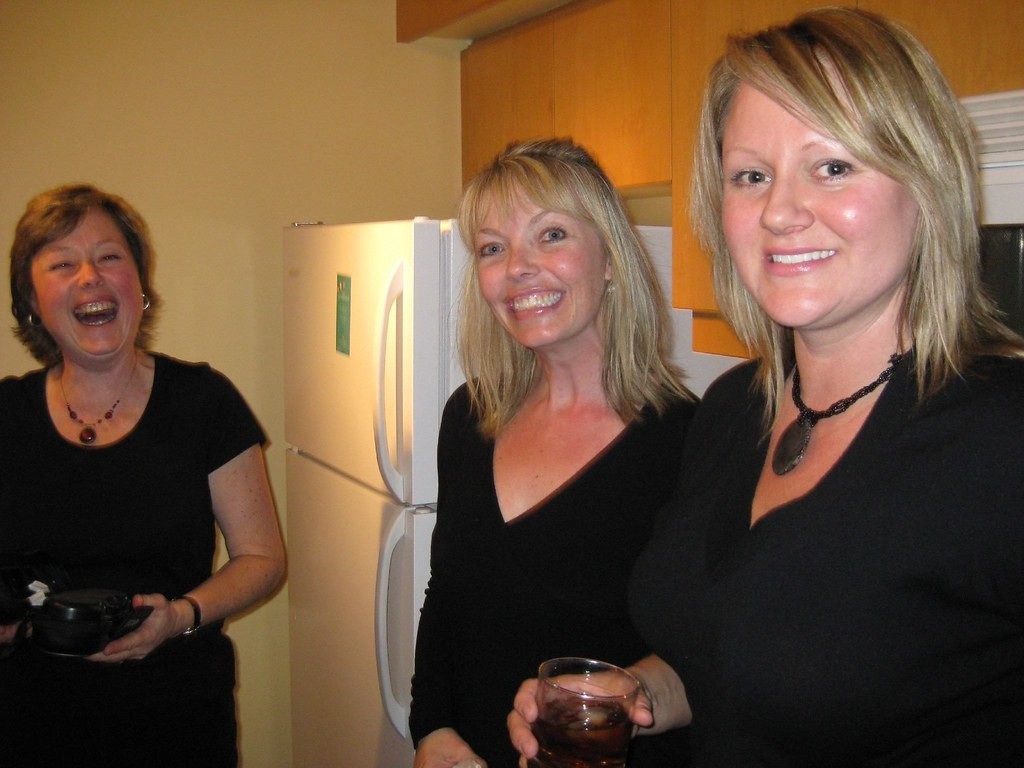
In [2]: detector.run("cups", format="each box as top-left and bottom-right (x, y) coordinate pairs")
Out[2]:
(525, 658), (640, 768)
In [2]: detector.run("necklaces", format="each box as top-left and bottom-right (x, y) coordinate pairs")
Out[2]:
(60, 350), (138, 446)
(772, 352), (903, 476)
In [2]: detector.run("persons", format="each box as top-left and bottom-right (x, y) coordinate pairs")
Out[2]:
(407, 8), (1024, 768)
(0, 186), (288, 768)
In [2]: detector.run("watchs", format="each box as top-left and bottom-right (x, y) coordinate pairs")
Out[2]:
(172, 595), (202, 636)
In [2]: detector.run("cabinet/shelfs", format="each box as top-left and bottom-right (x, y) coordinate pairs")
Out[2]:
(395, 0), (1024, 361)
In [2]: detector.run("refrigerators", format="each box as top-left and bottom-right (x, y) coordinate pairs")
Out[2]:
(270, 218), (677, 768)
